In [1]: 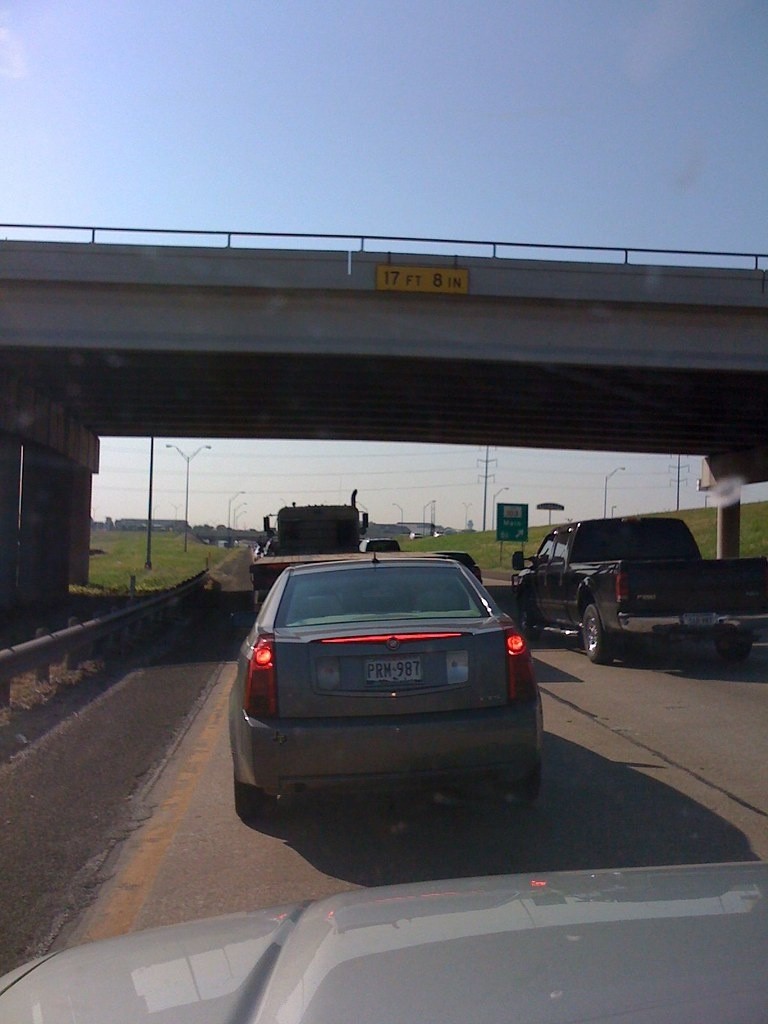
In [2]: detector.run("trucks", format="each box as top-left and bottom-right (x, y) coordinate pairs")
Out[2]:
(245, 487), (445, 615)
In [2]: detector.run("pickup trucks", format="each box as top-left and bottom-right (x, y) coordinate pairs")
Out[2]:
(510, 515), (768, 663)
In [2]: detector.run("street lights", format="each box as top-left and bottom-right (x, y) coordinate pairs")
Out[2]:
(422, 500), (435, 536)
(604, 466), (626, 520)
(392, 503), (403, 533)
(166, 443), (212, 551)
(228, 491), (246, 528)
(611, 505), (617, 519)
(233, 503), (248, 529)
(462, 502), (472, 529)
(491, 487), (509, 530)
(236, 511), (248, 528)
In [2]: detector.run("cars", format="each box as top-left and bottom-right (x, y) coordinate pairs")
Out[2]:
(409, 531), (422, 541)
(427, 550), (483, 586)
(358, 537), (401, 554)
(226, 555), (545, 819)
(433, 530), (444, 538)
